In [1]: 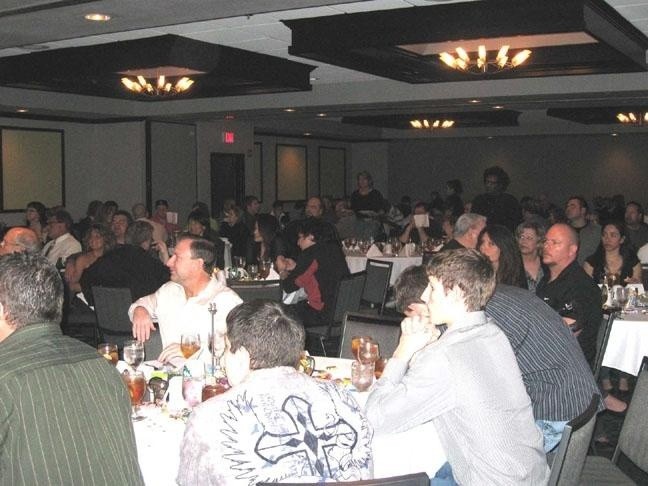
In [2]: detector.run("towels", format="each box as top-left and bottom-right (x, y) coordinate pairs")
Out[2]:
(365, 242), (416, 257)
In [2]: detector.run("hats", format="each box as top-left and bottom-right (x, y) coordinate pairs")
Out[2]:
(156, 200), (167, 205)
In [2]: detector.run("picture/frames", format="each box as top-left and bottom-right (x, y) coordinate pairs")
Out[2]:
(319, 146), (346, 201)
(276, 144), (308, 204)
(1, 126), (65, 213)
(244, 142), (262, 204)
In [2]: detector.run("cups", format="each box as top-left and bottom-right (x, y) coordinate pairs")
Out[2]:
(349, 335), (389, 389)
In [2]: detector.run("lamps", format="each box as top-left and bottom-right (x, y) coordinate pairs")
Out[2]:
(408, 118), (455, 132)
(120, 68), (197, 98)
(438, 44), (533, 78)
(617, 111), (648, 125)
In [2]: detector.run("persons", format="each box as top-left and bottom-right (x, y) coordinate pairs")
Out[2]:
(2, 167), (648, 486)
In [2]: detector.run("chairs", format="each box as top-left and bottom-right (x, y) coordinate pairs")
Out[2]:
(579, 355), (648, 485)
(226, 280), (284, 310)
(62, 277), (97, 348)
(304, 269), (367, 357)
(588, 308), (614, 457)
(357, 258), (393, 316)
(546, 392), (600, 486)
(87, 279), (135, 358)
(256, 472), (431, 486)
(337, 310), (401, 360)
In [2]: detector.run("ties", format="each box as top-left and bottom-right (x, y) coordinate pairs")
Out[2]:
(44, 239), (55, 257)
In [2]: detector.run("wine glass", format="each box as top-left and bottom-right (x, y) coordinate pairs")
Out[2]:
(97, 331), (314, 422)
(343, 236), (438, 256)
(603, 275), (648, 315)
(232, 256), (271, 282)
